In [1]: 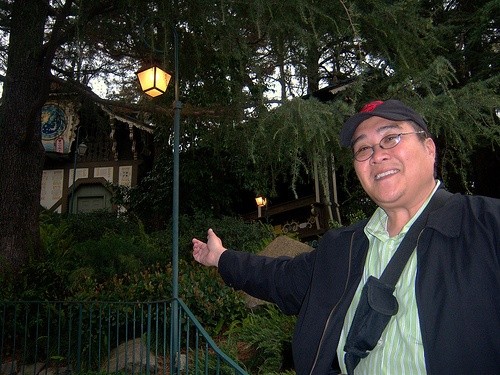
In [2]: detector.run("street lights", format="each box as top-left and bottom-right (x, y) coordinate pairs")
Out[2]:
(131, 13), (180, 374)
(254, 192), (270, 224)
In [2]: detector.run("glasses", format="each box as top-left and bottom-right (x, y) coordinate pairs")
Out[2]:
(351, 131), (427, 162)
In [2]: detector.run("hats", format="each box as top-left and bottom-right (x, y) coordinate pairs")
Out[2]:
(341, 100), (428, 147)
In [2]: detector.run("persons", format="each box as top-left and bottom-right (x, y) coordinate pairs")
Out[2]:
(192, 99), (500, 375)
